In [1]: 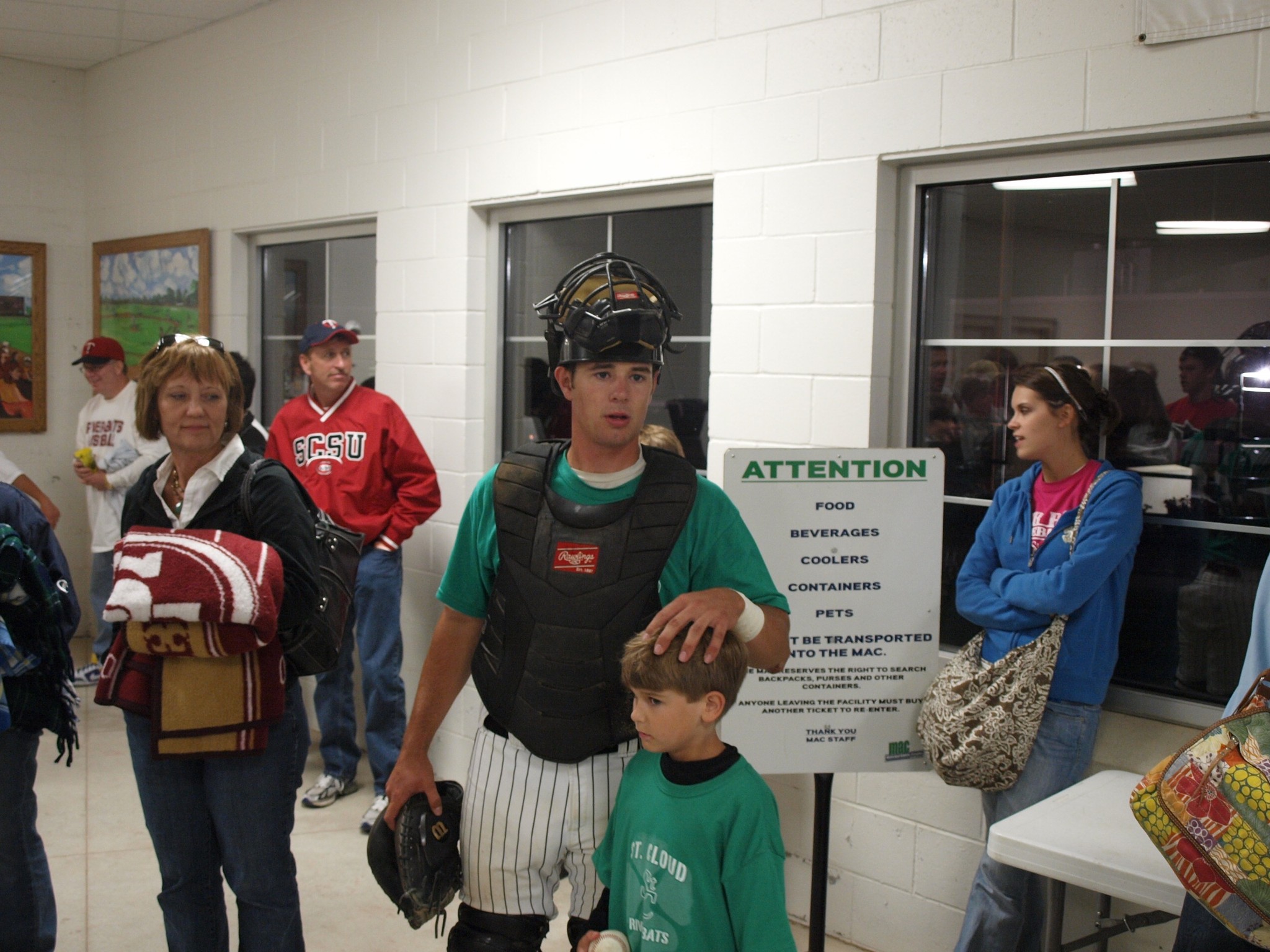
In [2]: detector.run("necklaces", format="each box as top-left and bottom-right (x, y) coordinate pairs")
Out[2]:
(170, 465), (185, 514)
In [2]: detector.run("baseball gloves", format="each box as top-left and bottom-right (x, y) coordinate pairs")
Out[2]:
(367, 780), (464, 939)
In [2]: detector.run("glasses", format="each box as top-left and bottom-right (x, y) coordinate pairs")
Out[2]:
(79, 361), (118, 374)
(148, 333), (225, 360)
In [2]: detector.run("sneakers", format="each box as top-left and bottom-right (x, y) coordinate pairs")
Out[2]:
(301, 774), (357, 807)
(73, 661), (102, 686)
(360, 793), (389, 834)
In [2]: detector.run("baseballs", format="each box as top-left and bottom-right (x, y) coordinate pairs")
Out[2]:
(588, 929), (631, 952)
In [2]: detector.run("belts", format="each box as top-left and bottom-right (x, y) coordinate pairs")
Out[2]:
(485, 714), (618, 754)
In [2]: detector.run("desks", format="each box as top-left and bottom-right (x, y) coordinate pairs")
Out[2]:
(986, 771), (1186, 952)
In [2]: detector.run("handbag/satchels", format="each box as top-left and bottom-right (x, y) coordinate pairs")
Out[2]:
(918, 620), (1065, 793)
(241, 458), (365, 676)
(1130, 668), (1270, 950)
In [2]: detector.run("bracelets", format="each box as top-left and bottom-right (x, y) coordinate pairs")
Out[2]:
(728, 586), (766, 643)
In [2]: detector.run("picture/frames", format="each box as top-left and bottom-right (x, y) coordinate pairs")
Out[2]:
(1, 239), (46, 433)
(92, 226), (212, 371)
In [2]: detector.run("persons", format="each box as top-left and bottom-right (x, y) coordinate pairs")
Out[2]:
(270, 320), (441, 812)
(101, 333), (321, 952)
(382, 250), (791, 951)
(953, 363), (1142, 952)
(70, 334), (171, 689)
(638, 424), (684, 460)
(576, 622), (798, 951)
(918, 356), (1168, 472)
(0, 450), (82, 952)
(1163, 347), (1240, 469)
(1169, 547), (1270, 952)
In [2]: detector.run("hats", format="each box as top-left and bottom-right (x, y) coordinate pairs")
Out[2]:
(298, 319), (359, 355)
(72, 336), (124, 365)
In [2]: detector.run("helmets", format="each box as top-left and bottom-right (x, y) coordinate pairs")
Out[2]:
(534, 252), (684, 365)
